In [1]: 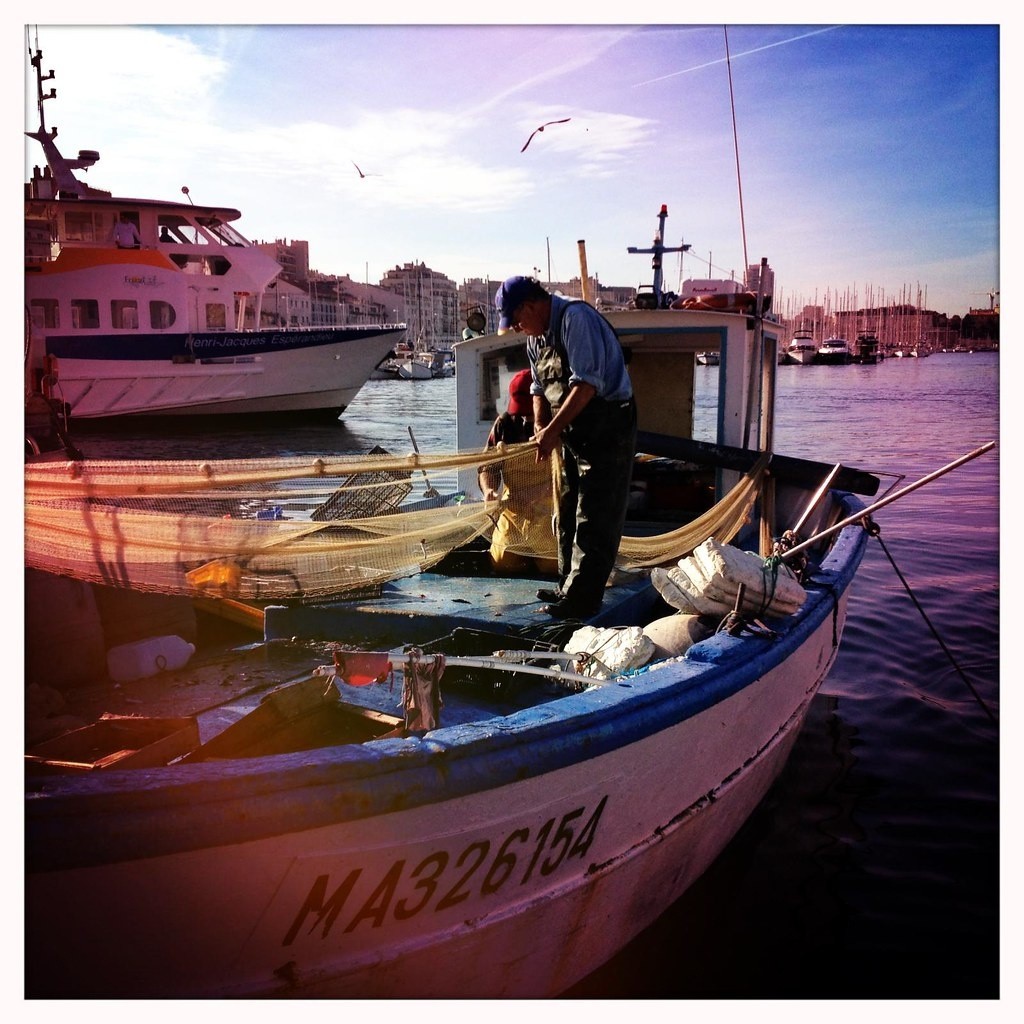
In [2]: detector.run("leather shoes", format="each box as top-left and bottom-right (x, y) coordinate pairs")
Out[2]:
(536, 588), (600, 616)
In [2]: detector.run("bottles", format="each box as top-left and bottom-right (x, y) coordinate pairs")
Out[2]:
(106, 632), (196, 682)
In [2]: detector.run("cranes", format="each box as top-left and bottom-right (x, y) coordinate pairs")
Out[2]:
(965, 290), (1000, 311)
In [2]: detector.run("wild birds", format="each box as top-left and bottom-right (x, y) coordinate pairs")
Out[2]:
(351, 160), (385, 179)
(519, 116), (572, 155)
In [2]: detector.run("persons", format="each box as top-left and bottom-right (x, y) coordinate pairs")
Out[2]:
(478, 276), (637, 620)
(159, 226), (176, 243)
(112, 213), (140, 248)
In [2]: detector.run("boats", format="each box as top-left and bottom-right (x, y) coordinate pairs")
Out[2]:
(25, 24), (410, 418)
(27, 204), (880, 1000)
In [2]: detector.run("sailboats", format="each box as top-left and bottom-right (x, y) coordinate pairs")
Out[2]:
(365, 248), (979, 382)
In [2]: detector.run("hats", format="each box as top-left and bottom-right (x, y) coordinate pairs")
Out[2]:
(506, 368), (535, 412)
(494, 275), (540, 336)
